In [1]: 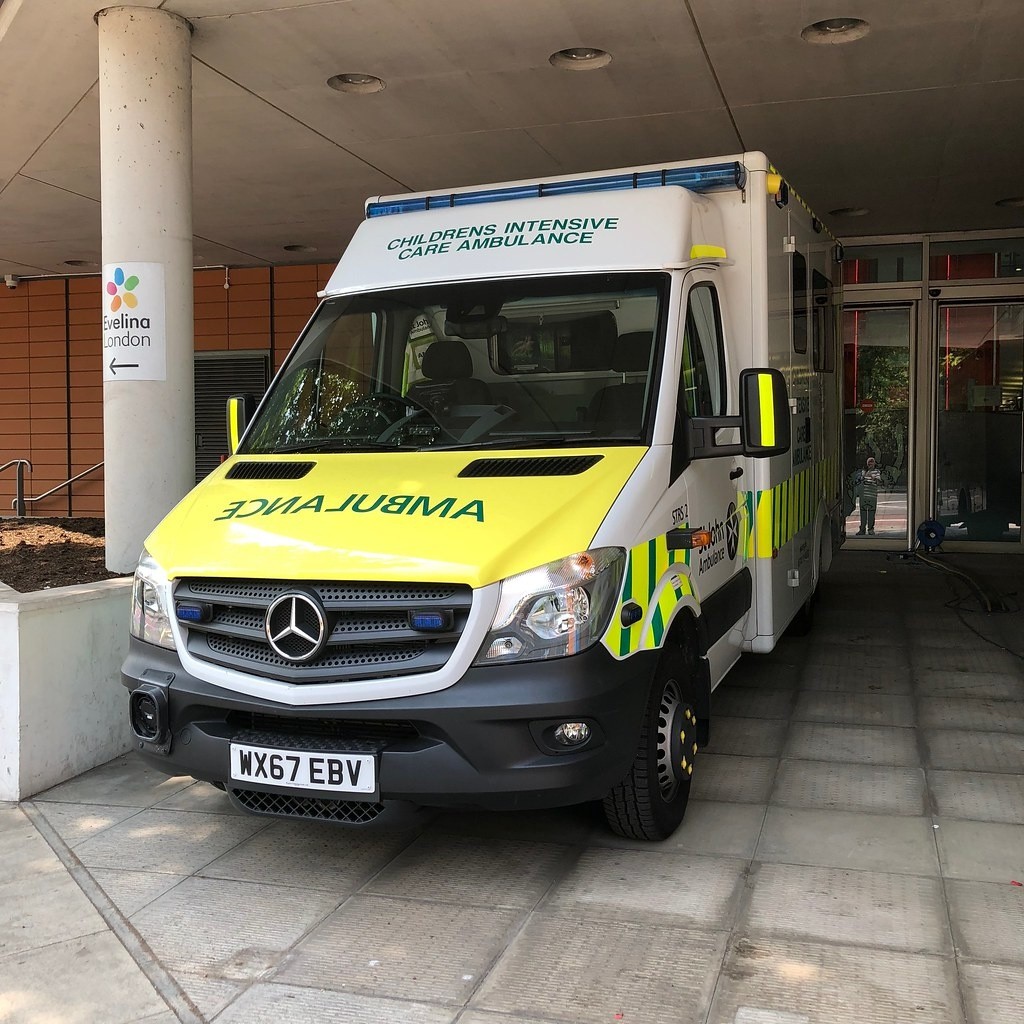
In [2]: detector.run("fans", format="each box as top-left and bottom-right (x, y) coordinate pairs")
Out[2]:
(917, 521), (945, 554)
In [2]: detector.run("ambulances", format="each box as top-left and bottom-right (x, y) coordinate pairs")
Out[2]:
(115, 148), (849, 843)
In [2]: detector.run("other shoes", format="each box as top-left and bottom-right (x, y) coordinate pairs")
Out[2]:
(868, 529), (875, 536)
(856, 528), (865, 535)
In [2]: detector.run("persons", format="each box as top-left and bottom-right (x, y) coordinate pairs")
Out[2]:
(855, 457), (880, 537)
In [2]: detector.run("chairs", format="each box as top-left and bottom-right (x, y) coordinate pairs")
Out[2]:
(402, 341), (492, 429)
(576, 332), (682, 434)
